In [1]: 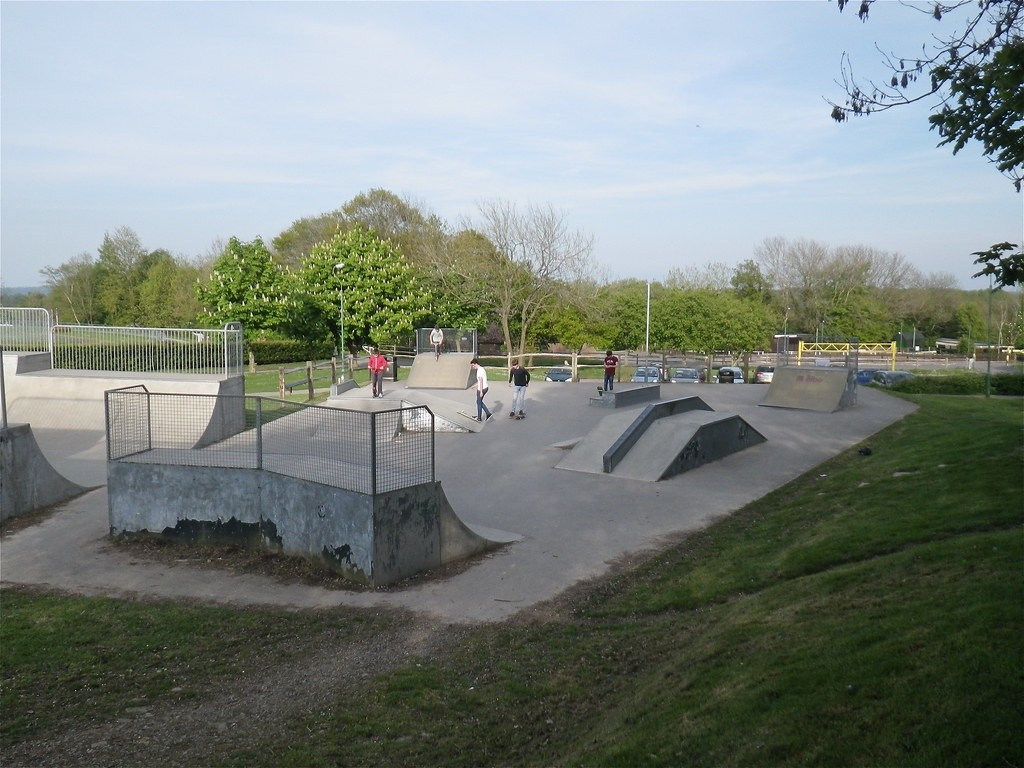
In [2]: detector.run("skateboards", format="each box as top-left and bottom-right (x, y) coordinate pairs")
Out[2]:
(597, 386), (604, 396)
(514, 413), (527, 420)
(456, 409), (481, 424)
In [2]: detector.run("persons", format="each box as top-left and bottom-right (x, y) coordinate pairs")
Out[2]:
(470, 358), (494, 423)
(603, 350), (618, 392)
(698, 371), (706, 383)
(429, 324), (444, 356)
(368, 348), (387, 399)
(968, 357), (974, 370)
(507, 359), (531, 419)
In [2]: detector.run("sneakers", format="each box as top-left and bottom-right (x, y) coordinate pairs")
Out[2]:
(484, 413), (493, 422)
(476, 418), (481, 422)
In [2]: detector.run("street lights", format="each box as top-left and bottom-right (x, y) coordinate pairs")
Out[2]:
(332, 263), (345, 383)
(821, 320), (825, 342)
(646, 283), (650, 353)
(783, 307), (790, 366)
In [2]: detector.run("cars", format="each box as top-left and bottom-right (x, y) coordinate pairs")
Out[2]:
(670, 367), (700, 383)
(752, 365), (775, 383)
(545, 367), (580, 383)
(713, 365), (744, 384)
(631, 361), (668, 384)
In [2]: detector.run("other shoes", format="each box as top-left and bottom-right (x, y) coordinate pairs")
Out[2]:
(379, 392), (382, 398)
(518, 410), (523, 416)
(509, 412), (515, 417)
